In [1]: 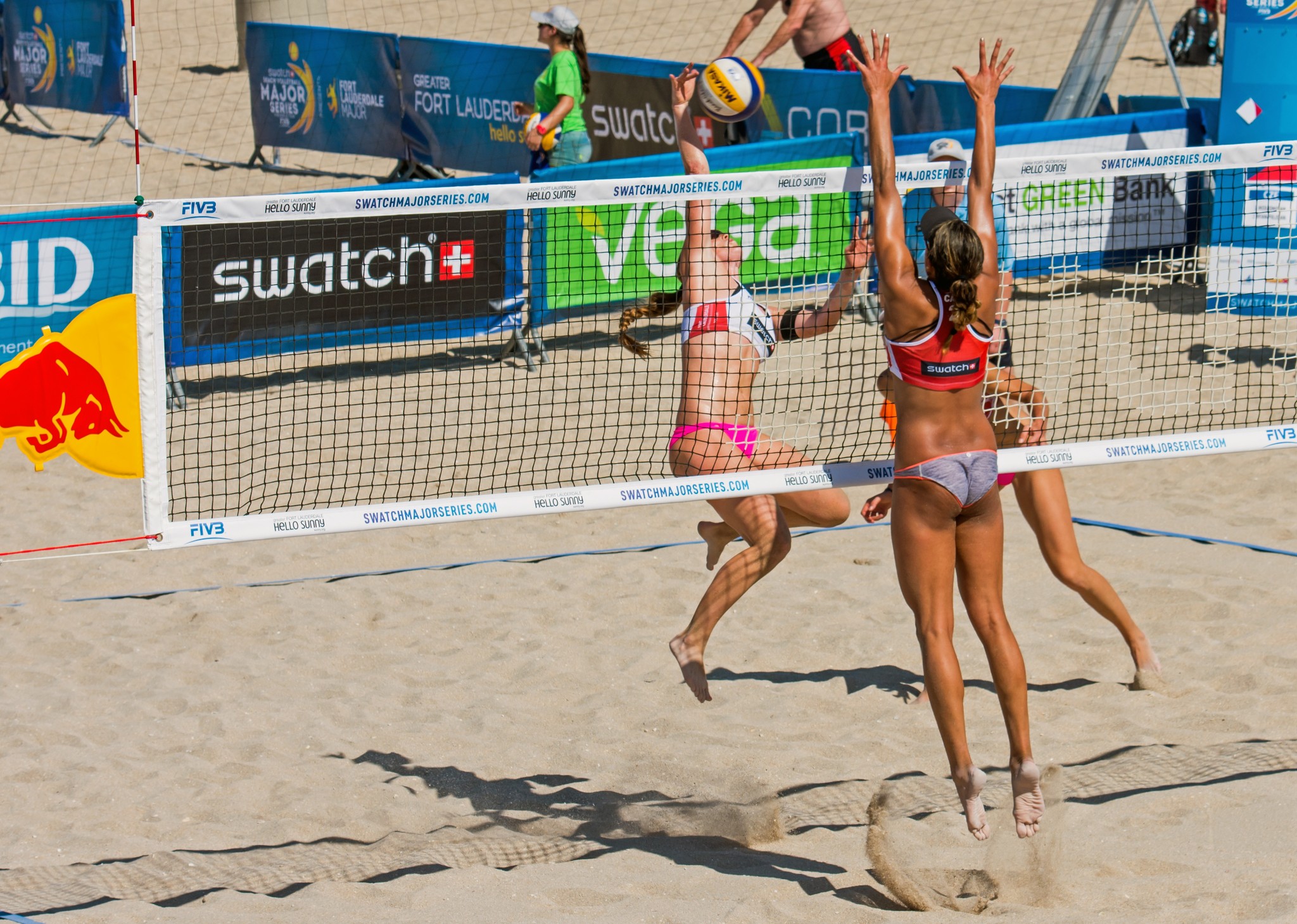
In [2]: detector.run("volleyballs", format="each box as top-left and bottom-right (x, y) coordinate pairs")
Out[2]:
(523, 112), (563, 152)
(697, 56), (766, 124)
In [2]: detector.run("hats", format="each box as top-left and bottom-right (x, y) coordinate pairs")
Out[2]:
(927, 137), (964, 162)
(530, 6), (578, 35)
(920, 205), (984, 282)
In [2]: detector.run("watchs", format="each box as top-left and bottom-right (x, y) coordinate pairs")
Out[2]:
(995, 318), (1007, 327)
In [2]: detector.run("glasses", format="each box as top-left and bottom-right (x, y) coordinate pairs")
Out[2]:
(710, 230), (722, 239)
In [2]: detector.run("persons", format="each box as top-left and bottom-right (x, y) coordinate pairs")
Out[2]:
(513, 6), (592, 174)
(712, 0), (868, 72)
(867, 139), (1015, 377)
(846, 29), (1044, 838)
(860, 360), (1160, 704)
(619, 63), (874, 702)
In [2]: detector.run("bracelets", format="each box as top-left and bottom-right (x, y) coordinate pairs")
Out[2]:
(536, 125), (547, 135)
(885, 487), (893, 493)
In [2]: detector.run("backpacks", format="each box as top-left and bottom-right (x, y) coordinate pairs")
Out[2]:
(1168, 6), (1221, 67)
